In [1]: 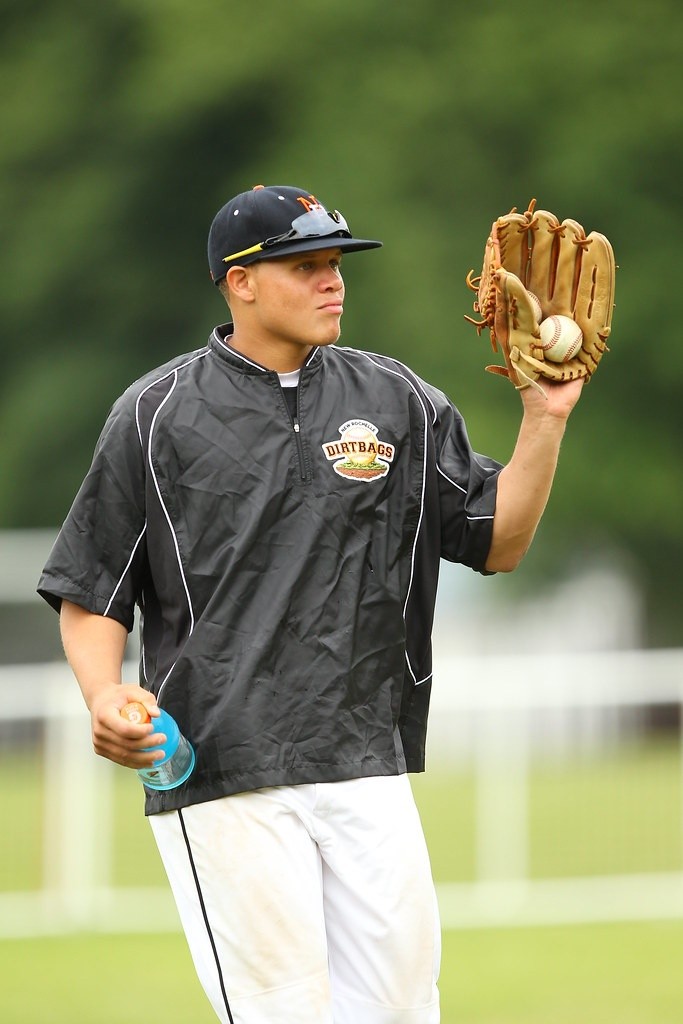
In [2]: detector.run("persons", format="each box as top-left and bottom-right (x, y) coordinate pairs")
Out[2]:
(36, 185), (616, 1024)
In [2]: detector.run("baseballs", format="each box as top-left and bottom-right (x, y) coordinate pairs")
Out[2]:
(539, 314), (583, 363)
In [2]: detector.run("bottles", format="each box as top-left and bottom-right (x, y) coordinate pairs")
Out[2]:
(121, 702), (195, 790)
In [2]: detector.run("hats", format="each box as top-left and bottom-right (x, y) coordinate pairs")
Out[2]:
(207, 184), (384, 285)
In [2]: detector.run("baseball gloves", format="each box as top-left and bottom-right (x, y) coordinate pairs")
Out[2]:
(459, 193), (620, 394)
